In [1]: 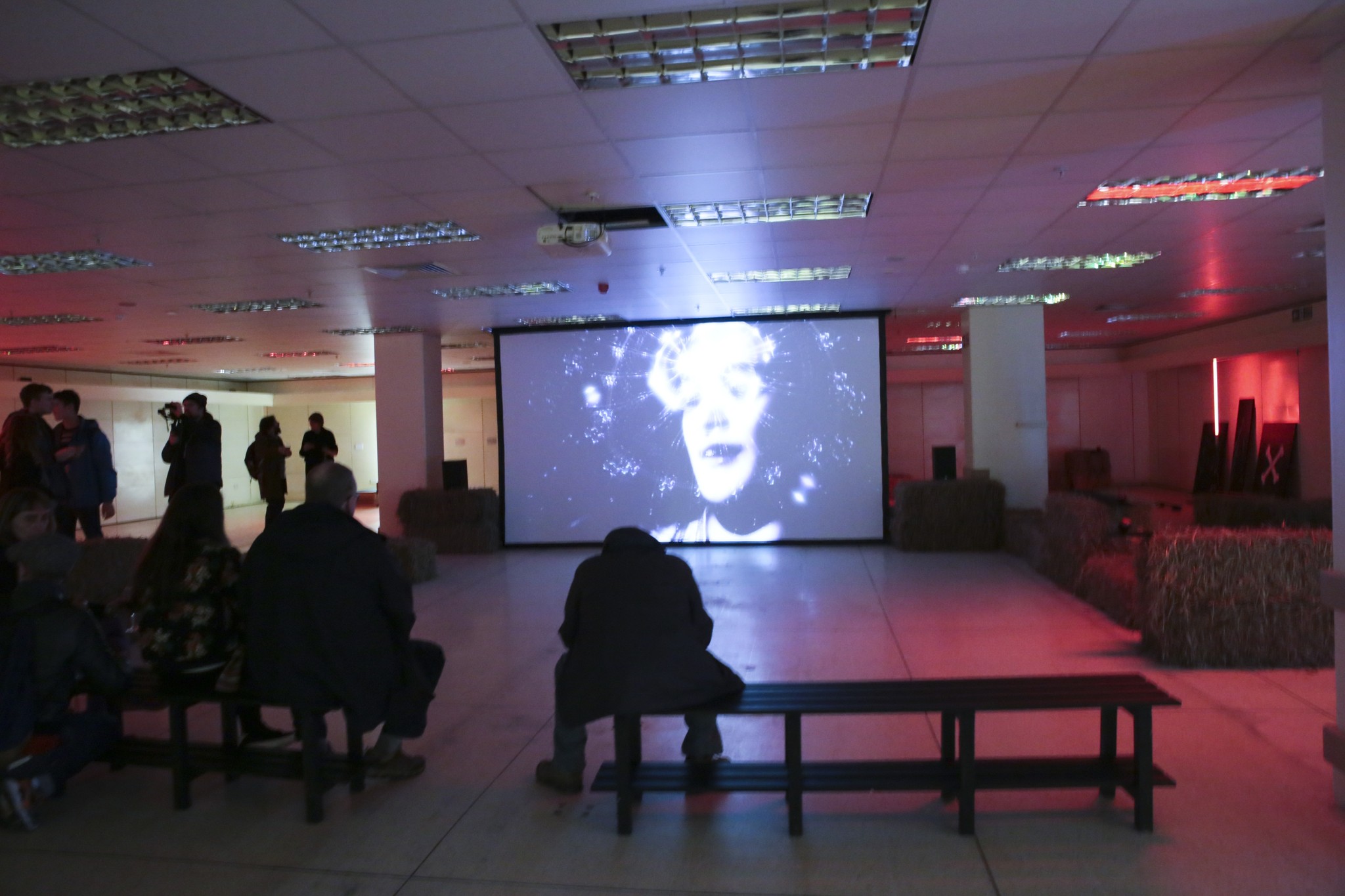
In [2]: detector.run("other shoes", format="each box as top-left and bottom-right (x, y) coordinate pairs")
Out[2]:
(239, 727), (299, 752)
(684, 755), (731, 763)
(362, 746), (428, 778)
(534, 759), (582, 789)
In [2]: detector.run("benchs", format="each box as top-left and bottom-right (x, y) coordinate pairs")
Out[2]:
(60, 675), (365, 827)
(590, 673), (1181, 837)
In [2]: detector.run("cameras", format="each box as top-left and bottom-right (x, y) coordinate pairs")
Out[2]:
(158, 403), (178, 421)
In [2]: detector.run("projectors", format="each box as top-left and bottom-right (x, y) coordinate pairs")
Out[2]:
(535, 223), (612, 258)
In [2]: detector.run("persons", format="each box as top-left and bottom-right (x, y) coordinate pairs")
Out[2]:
(255, 416), (290, 526)
(240, 463), (443, 775)
(0, 383), (63, 488)
(639, 321), (840, 542)
(535, 526), (743, 792)
(49, 388), (118, 540)
(1, 491), (56, 594)
(161, 393), (222, 505)
(0, 535), (123, 829)
(129, 484), (300, 749)
(299, 412), (337, 476)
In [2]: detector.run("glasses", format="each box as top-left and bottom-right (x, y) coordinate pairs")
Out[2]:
(348, 491), (360, 503)
